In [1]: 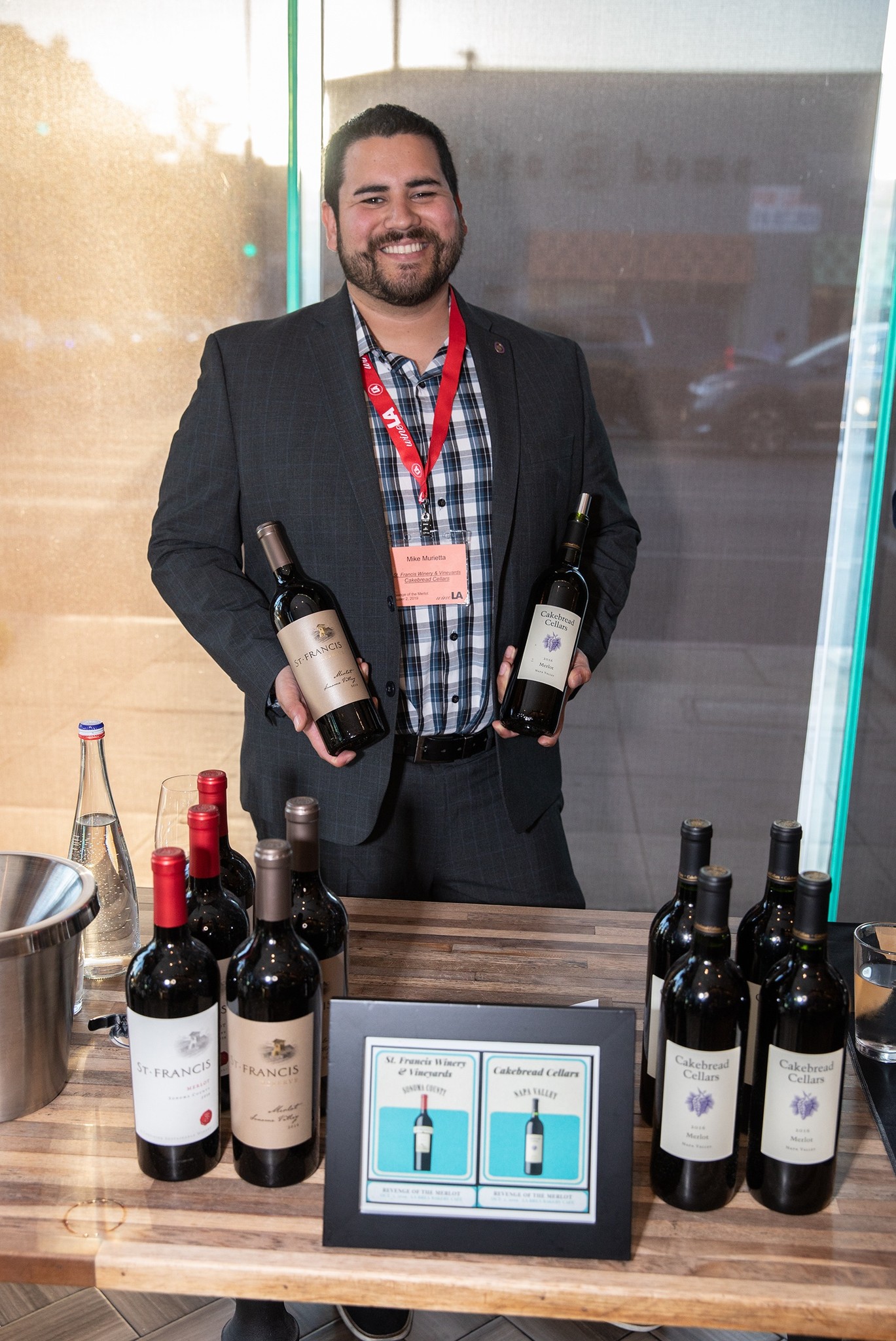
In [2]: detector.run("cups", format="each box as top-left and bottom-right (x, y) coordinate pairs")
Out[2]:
(853, 922), (896, 1063)
(155, 774), (201, 865)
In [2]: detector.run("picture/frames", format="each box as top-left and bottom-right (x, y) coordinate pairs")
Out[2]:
(321, 996), (637, 1262)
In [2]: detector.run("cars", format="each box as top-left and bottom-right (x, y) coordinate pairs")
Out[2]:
(682, 313), (887, 459)
(516, 304), (663, 432)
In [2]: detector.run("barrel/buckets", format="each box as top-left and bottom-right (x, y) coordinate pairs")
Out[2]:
(0, 851), (101, 1123)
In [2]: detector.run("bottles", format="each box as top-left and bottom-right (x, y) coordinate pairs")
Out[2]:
(256, 519), (387, 755)
(272, 796), (351, 1117)
(125, 847), (220, 1182)
(180, 770), (256, 1110)
(500, 492), (592, 736)
(525, 1098), (544, 1175)
(67, 722), (140, 1017)
(638, 820), (851, 1214)
(225, 839), (321, 1186)
(413, 1095), (433, 1171)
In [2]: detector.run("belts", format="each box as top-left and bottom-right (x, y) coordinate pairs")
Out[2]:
(393, 726), (497, 765)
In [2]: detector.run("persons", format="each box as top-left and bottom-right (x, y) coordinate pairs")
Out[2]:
(148, 103), (642, 1340)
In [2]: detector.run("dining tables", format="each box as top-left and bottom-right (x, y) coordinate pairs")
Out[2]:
(0, 886), (896, 1341)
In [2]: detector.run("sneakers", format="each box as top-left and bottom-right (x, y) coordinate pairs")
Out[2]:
(336, 1303), (414, 1341)
(604, 1320), (663, 1333)
